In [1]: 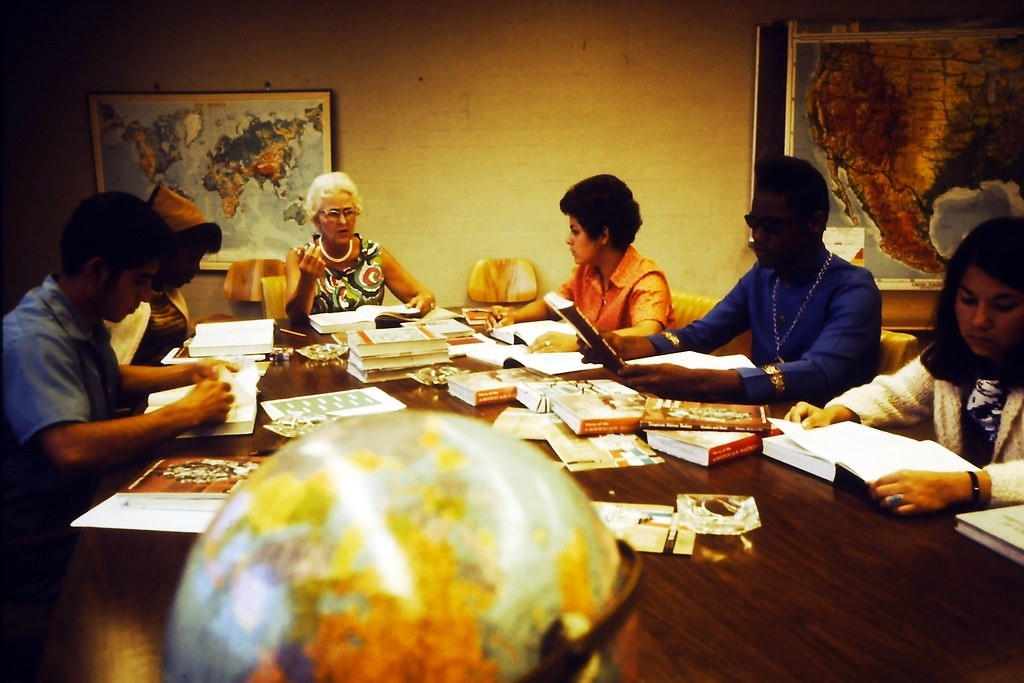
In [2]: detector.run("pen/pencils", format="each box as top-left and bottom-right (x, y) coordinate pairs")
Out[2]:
(324, 267), (333, 277)
(280, 328), (308, 337)
(249, 448), (275, 458)
(500, 313), (508, 317)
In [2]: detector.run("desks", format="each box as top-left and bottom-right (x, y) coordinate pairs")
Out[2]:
(20, 309), (1024, 683)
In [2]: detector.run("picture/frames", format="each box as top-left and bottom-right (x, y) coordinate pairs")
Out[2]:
(91, 87), (337, 274)
(747, 22), (1024, 295)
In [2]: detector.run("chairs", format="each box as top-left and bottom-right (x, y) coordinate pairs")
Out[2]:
(259, 276), (289, 322)
(192, 259), (288, 331)
(466, 256), (539, 309)
(663, 289), (753, 369)
(875, 328), (921, 376)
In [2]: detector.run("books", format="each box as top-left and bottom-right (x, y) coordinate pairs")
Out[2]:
(955, 505), (1024, 564)
(446, 291), (782, 472)
(144, 318), (293, 438)
(763, 417), (981, 484)
(116, 456), (271, 499)
(308, 304), (464, 333)
(591, 502), (696, 554)
(331, 319), (496, 383)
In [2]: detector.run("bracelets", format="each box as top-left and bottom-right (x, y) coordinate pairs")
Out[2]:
(967, 472), (979, 506)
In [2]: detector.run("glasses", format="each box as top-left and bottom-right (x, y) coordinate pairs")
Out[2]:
(744, 214), (811, 233)
(318, 207), (360, 220)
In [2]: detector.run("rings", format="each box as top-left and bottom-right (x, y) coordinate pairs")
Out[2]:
(545, 342), (549, 346)
(885, 495), (903, 504)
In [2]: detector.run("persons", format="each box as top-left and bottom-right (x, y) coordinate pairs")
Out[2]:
(577, 157), (883, 405)
(1, 194), (241, 602)
(784, 216), (1024, 514)
(285, 170), (435, 318)
(485, 174), (676, 353)
(108, 185), (222, 365)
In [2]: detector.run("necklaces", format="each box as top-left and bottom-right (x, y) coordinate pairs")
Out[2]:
(152, 291), (163, 295)
(772, 253), (833, 363)
(317, 235), (353, 262)
